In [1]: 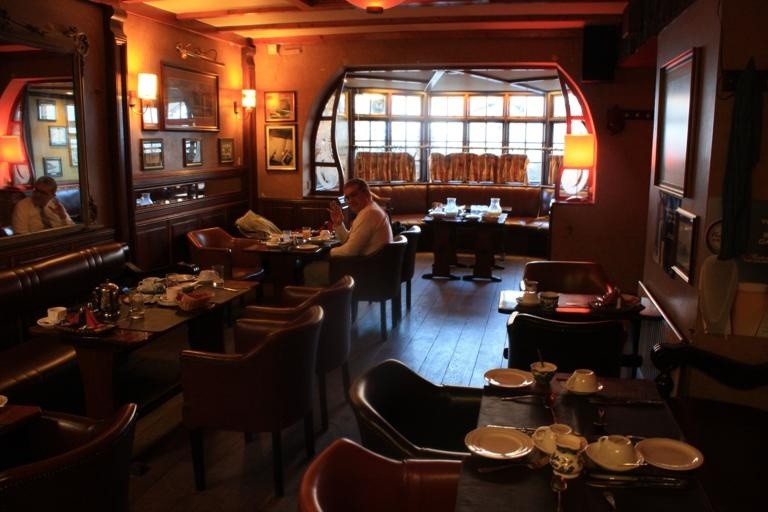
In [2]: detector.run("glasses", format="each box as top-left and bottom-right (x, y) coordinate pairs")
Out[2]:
(344, 191), (359, 201)
(33, 187), (48, 196)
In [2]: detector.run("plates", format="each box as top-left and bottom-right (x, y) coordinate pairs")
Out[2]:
(296, 245), (319, 249)
(560, 381), (603, 397)
(484, 368), (534, 388)
(159, 299), (180, 306)
(168, 274), (195, 282)
(37, 317), (66, 328)
(517, 301), (541, 307)
(464, 425), (704, 471)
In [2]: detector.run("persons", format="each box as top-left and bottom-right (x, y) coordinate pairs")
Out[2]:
(302, 178), (393, 287)
(11, 176), (75, 235)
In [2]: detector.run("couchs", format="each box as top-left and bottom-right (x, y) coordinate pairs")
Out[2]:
(425, 182), (548, 256)
(0, 240), (144, 407)
(359, 184), (428, 252)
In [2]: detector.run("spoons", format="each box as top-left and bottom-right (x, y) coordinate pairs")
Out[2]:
(551, 476), (567, 512)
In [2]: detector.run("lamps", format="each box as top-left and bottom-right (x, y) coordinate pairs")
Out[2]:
(233, 88), (257, 122)
(561, 133), (594, 203)
(0, 136), (26, 187)
(128, 72), (158, 117)
(350, 1), (402, 14)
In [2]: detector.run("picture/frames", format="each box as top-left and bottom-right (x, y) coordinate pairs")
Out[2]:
(263, 124), (297, 173)
(140, 137), (165, 170)
(183, 136), (203, 167)
(159, 59), (221, 132)
(66, 104), (77, 136)
(68, 137), (79, 167)
(48, 125), (68, 146)
(42, 156), (63, 176)
(264, 90), (296, 123)
(669, 205), (699, 285)
(218, 137), (236, 165)
(653, 45), (701, 198)
(37, 98), (56, 121)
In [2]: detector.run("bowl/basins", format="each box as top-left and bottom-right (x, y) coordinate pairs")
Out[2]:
(531, 362), (557, 385)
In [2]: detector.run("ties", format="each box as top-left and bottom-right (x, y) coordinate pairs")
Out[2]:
(39, 205), (53, 230)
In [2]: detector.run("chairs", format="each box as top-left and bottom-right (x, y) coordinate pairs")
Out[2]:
(518, 258), (607, 295)
(247, 275), (355, 432)
(0, 401), (138, 512)
(507, 308), (628, 377)
(185, 225), (264, 329)
(401, 223), (422, 312)
(329, 232), (409, 343)
(300, 436), (461, 512)
(0, 188), (25, 229)
(179, 303), (324, 496)
(347, 356), (482, 459)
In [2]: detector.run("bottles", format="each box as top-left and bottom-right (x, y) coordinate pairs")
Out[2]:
(488, 198), (502, 216)
(445, 198), (458, 218)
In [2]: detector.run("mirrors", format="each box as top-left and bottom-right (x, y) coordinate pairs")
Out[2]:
(0, 28), (99, 251)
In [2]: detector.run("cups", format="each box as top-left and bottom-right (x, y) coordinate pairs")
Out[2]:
(128, 288), (144, 319)
(48, 307), (67, 323)
(167, 287), (182, 301)
(302, 227), (311, 238)
(213, 265), (224, 288)
(522, 294), (538, 303)
(320, 230), (330, 240)
(283, 230), (291, 242)
(567, 369), (598, 392)
